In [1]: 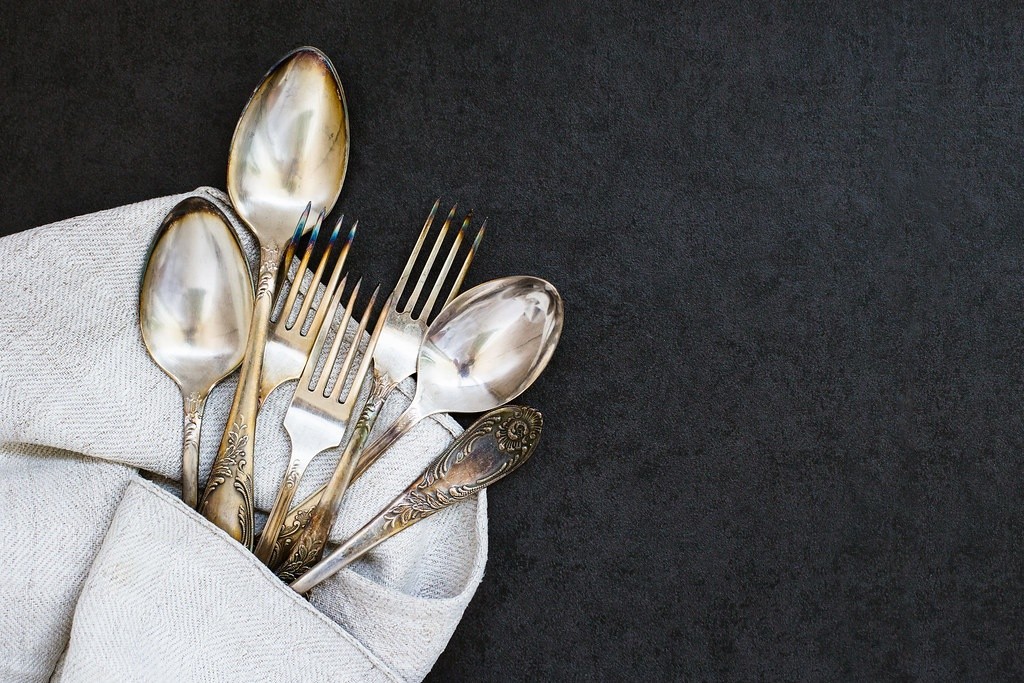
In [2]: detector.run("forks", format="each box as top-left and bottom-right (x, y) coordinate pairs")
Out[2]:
(253, 199), (360, 422)
(253, 273), (396, 570)
(272, 193), (491, 605)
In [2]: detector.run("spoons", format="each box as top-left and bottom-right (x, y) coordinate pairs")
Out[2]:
(194, 46), (351, 556)
(289, 404), (544, 596)
(253, 273), (566, 574)
(136, 195), (257, 509)
